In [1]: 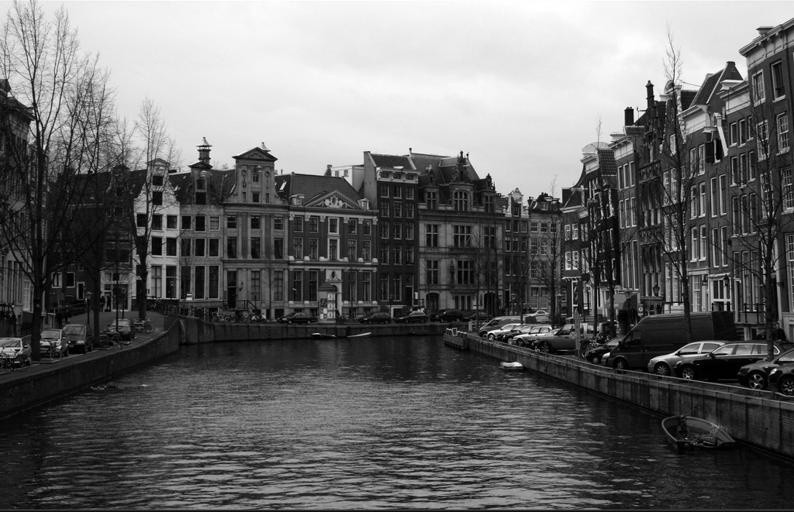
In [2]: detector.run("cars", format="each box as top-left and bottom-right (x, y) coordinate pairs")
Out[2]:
(646, 340), (729, 377)
(460, 312), (493, 322)
(738, 347), (794, 390)
(105, 317), (135, 342)
(276, 311), (318, 325)
(478, 310), (627, 364)
(769, 361), (794, 397)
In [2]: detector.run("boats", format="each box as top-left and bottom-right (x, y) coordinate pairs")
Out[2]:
(662, 413), (738, 453)
(311, 326), (373, 340)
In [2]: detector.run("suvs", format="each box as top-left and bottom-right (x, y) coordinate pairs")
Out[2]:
(40, 327), (72, 357)
(677, 342), (785, 383)
(64, 323), (94, 354)
(359, 312), (391, 324)
(394, 310), (428, 324)
(0, 336), (32, 365)
(430, 308), (463, 323)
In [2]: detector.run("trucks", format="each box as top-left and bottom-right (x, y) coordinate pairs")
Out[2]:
(605, 311), (738, 371)
